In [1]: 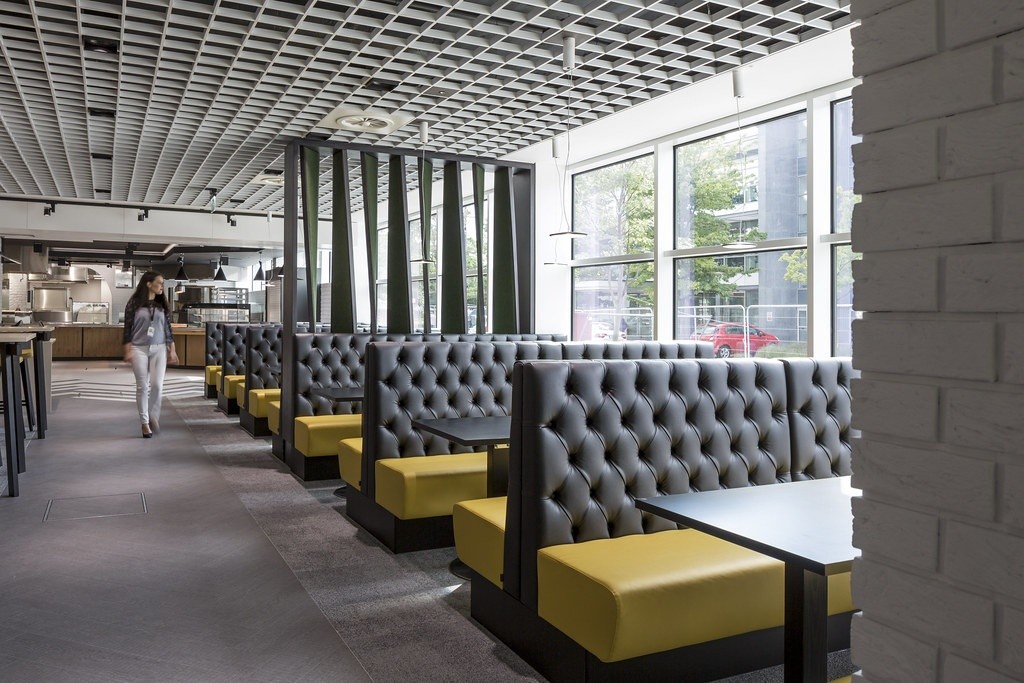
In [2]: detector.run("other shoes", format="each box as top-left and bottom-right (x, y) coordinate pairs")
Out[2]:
(142, 423), (152, 438)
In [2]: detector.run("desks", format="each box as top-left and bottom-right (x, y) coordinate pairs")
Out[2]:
(0, 324), (56, 496)
(311, 384), (365, 498)
(633, 474), (861, 683)
(410, 415), (512, 581)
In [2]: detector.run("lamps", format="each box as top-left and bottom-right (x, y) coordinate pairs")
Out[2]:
(254, 251), (265, 280)
(214, 253), (226, 280)
(43, 200), (58, 216)
(262, 211), (276, 286)
(175, 257), (188, 280)
(548, 38), (588, 239)
(58, 256), (65, 266)
(227, 214), (236, 226)
(33, 242), (42, 252)
(544, 137), (572, 267)
(123, 261), (131, 271)
(409, 120), (435, 263)
(138, 207), (151, 221)
(721, 68), (758, 249)
(126, 247), (134, 256)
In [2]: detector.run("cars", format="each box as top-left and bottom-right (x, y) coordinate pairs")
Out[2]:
(689, 322), (780, 359)
(591, 322), (628, 341)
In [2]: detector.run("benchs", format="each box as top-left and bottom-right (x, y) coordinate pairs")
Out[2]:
(203, 319), (861, 683)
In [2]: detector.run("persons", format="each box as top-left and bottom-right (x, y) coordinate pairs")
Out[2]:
(123, 271), (178, 436)
